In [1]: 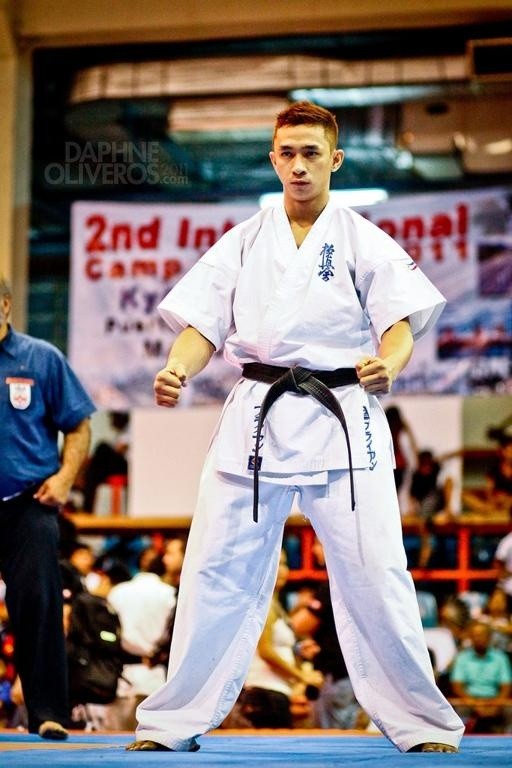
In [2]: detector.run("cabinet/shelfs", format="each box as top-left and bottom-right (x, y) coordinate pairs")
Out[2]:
(68, 513), (511, 640)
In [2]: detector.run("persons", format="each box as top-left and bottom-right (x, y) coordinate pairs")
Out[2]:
(460, 320), (489, 355)
(480, 321), (511, 356)
(0, 277), (100, 743)
(122, 98), (469, 757)
(435, 323), (464, 359)
